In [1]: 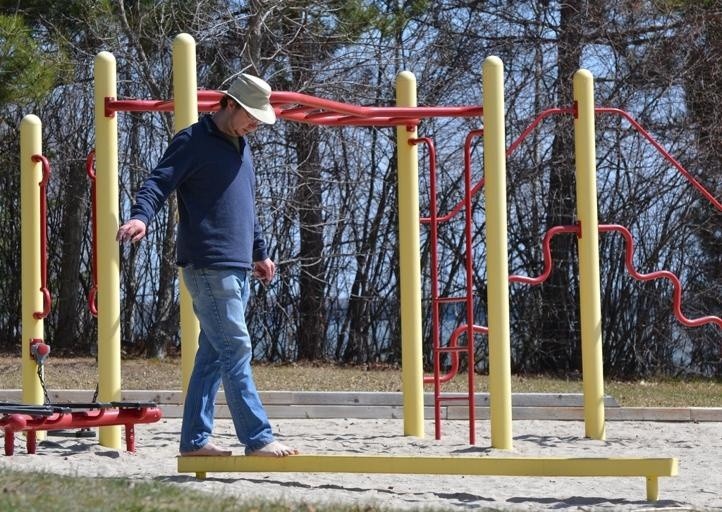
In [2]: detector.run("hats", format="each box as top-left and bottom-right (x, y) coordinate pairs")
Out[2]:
(221, 73), (276, 124)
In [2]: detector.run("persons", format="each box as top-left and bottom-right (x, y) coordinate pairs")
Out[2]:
(116, 74), (300, 457)
